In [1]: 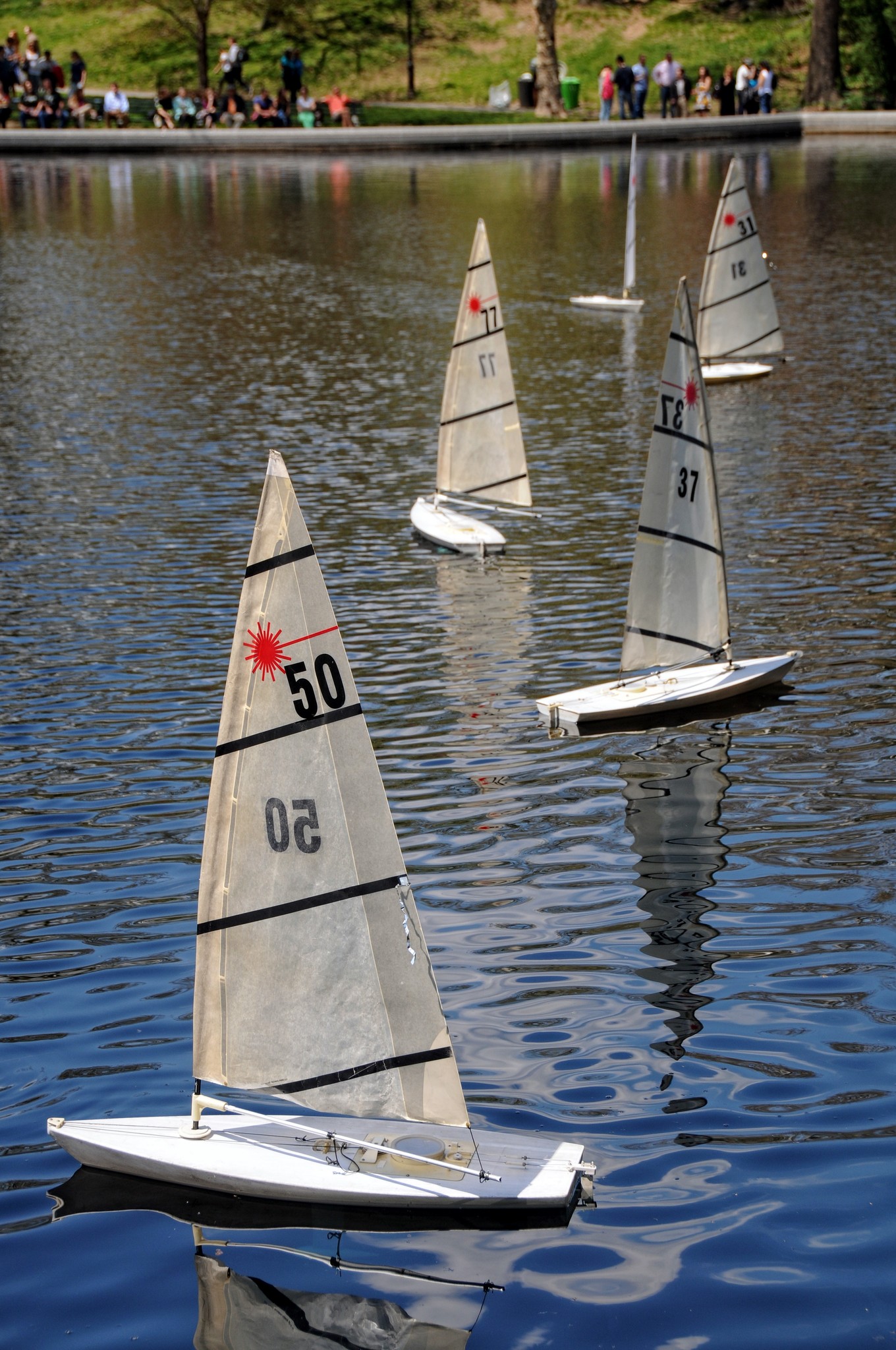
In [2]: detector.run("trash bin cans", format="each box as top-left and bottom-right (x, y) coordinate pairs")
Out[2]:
(517, 72), (538, 110)
(560, 76), (581, 110)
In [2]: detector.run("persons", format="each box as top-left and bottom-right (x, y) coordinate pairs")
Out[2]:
(598, 52), (779, 120)
(1, 24), (359, 127)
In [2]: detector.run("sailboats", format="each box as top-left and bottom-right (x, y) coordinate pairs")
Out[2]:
(408, 216), (542, 560)
(569, 132), (645, 314)
(693, 155), (785, 384)
(44, 447), (596, 1211)
(536, 275), (803, 724)
